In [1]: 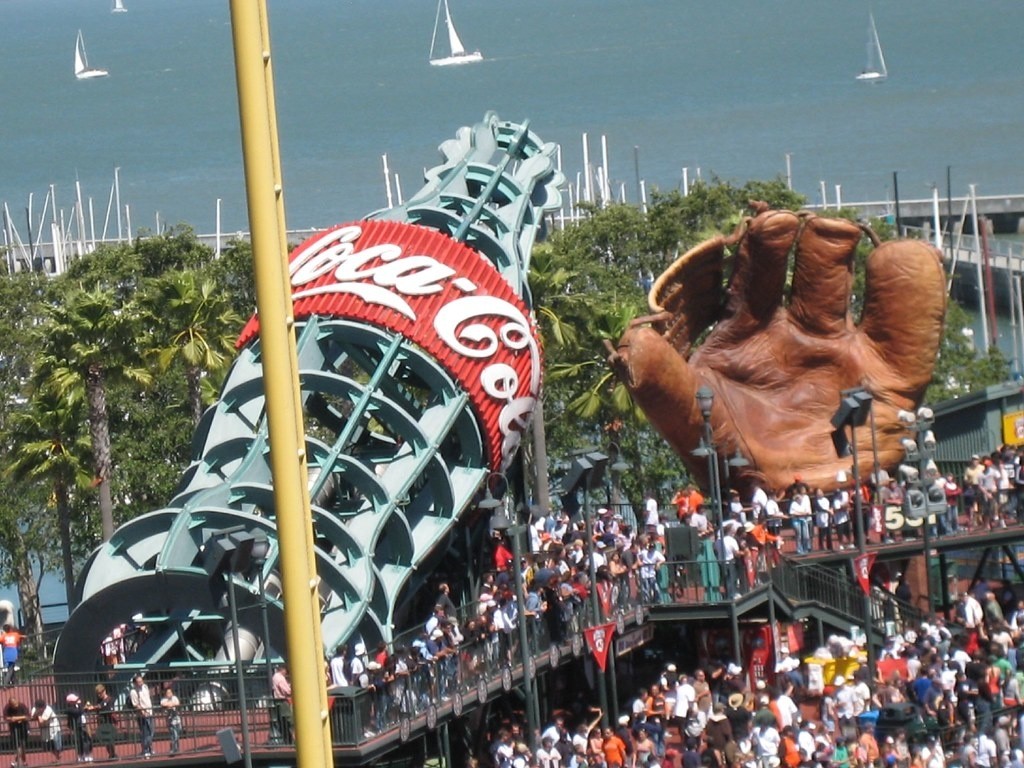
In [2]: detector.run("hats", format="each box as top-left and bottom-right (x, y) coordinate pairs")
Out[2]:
(95, 684), (105, 693)
(430, 630), (443, 640)
(714, 703), (727, 709)
(730, 666), (742, 675)
(367, 661), (381, 670)
(972, 454), (979, 460)
(596, 541), (606, 549)
(834, 676), (844, 686)
(66, 693), (79, 701)
(780, 647), (790, 654)
(355, 643), (365, 656)
(729, 693), (743, 707)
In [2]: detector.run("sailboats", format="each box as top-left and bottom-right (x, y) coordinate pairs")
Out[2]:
(110, 0), (127, 14)
(856, 14), (887, 79)
(429, 0), (482, 65)
(75, 29), (108, 79)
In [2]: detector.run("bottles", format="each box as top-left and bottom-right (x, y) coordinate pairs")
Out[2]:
(49, 112), (567, 715)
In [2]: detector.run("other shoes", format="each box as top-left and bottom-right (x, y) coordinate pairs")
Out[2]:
(10, 762), (28, 768)
(840, 546), (844, 550)
(144, 751), (156, 759)
(364, 731), (376, 738)
(848, 544), (855, 548)
(1000, 519), (1007, 528)
(78, 757), (93, 762)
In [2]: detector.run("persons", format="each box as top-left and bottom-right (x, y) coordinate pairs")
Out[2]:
(3, 697), (30, 768)
(30, 699), (63, 766)
(266, 442), (1024, 768)
(160, 687), (183, 756)
(0, 624), (28, 691)
(84, 683), (118, 759)
(65, 694), (94, 762)
(130, 676), (156, 760)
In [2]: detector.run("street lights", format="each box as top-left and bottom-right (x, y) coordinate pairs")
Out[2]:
(480, 472), (538, 768)
(562, 453), (611, 735)
(205, 524), (256, 768)
(248, 527), (285, 748)
(690, 387), (750, 598)
(830, 387), (875, 694)
(899, 407), (947, 627)
